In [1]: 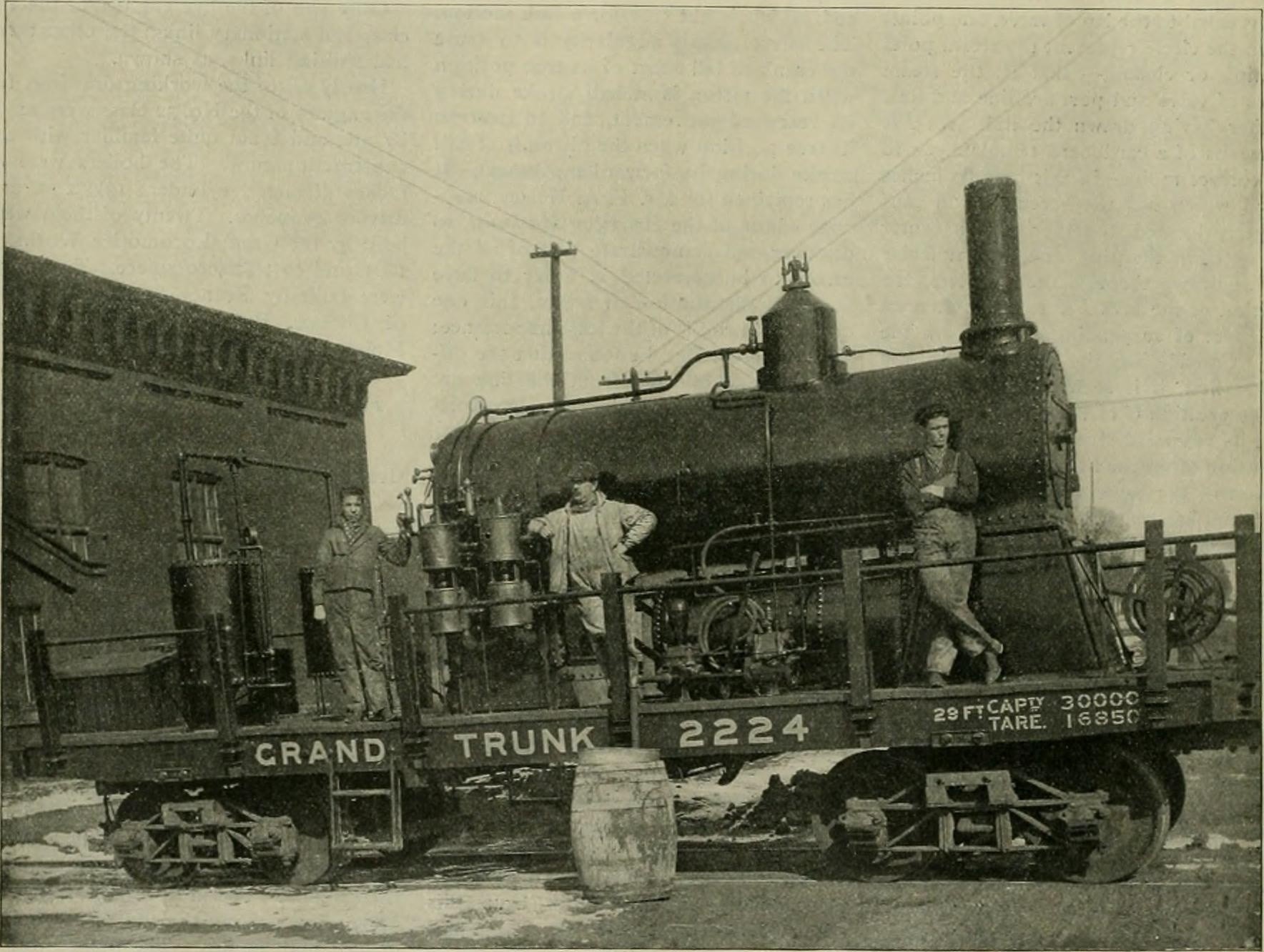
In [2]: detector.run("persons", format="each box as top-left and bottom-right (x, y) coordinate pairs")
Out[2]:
(310, 485), (411, 721)
(896, 401), (1004, 684)
(529, 461), (659, 697)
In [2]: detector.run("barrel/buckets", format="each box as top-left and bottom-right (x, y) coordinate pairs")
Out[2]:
(570, 747), (678, 904)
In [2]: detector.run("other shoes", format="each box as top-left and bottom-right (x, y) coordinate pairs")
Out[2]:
(375, 708), (398, 722)
(981, 638), (1005, 686)
(927, 672), (948, 689)
(345, 713), (364, 723)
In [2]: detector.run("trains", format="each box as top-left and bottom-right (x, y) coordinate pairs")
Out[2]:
(34, 177), (1264, 894)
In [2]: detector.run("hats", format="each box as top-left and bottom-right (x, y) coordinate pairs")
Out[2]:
(913, 403), (949, 424)
(567, 460), (599, 482)
